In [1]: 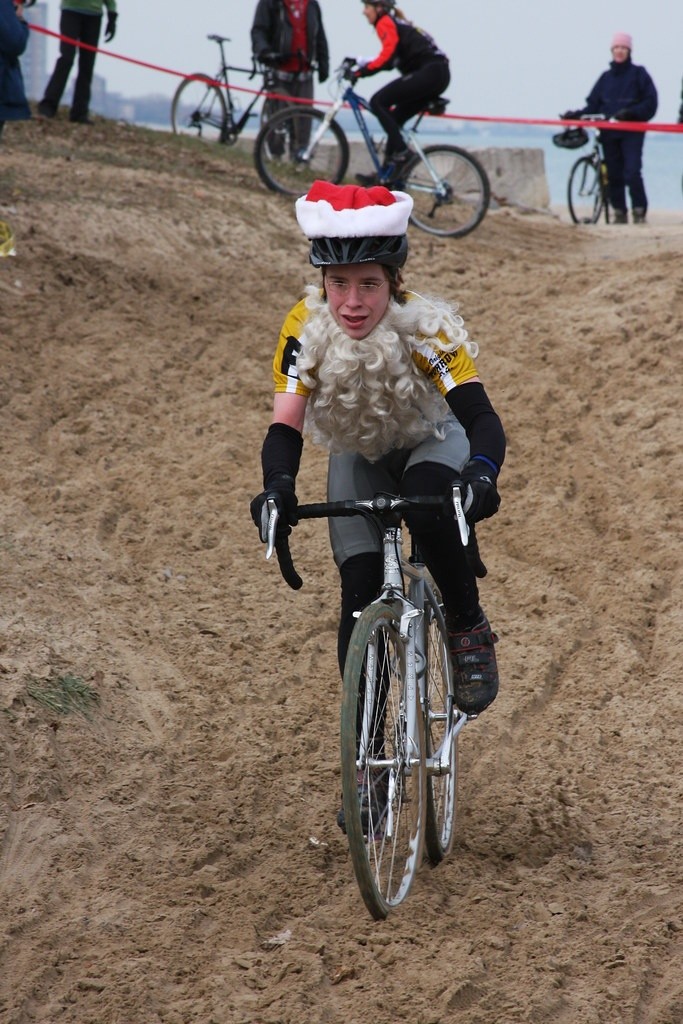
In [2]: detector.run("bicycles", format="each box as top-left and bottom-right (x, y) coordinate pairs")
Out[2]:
(171, 31), (306, 165)
(253, 59), (492, 240)
(263, 479), (486, 920)
(558, 113), (613, 225)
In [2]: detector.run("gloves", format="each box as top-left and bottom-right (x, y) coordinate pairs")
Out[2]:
(459, 454), (501, 524)
(318, 57), (328, 82)
(250, 463), (298, 543)
(105, 10), (118, 42)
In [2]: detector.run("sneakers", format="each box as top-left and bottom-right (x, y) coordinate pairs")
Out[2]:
(337, 753), (390, 834)
(356, 173), (406, 190)
(379, 148), (421, 186)
(446, 614), (500, 715)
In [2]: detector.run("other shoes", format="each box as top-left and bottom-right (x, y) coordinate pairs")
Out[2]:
(632, 208), (646, 224)
(613, 209), (628, 224)
(69, 113), (95, 125)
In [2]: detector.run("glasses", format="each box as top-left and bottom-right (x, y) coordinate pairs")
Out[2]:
(325, 272), (388, 294)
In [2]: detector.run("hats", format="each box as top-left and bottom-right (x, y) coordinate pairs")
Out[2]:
(295, 180), (414, 238)
(611, 32), (632, 51)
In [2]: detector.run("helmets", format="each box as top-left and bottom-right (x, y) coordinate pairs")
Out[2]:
(554, 129), (588, 148)
(309, 238), (408, 268)
(363, 0), (395, 8)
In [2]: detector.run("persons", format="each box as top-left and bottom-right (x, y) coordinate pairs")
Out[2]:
(250, 180), (507, 839)
(0, 0), (118, 143)
(251, 0), (330, 165)
(564, 33), (658, 224)
(352, 0), (450, 188)
